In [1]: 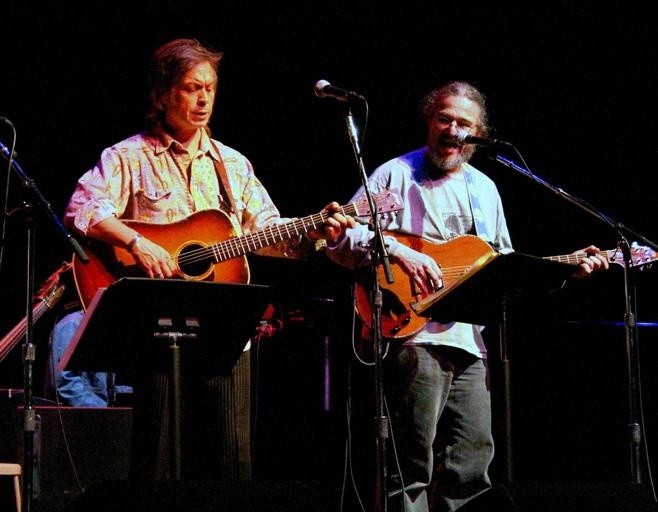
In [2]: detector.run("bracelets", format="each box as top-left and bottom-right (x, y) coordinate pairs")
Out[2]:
(125, 233), (141, 252)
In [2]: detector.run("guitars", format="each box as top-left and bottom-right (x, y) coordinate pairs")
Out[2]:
(62, 188), (405, 314)
(352, 230), (658, 340)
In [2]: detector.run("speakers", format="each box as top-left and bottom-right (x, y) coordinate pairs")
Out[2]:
(456, 481), (658, 512)
(0, 390), (62, 512)
(63, 478), (287, 512)
(17, 406), (136, 512)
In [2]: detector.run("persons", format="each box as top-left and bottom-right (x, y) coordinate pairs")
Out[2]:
(44, 308), (132, 409)
(326, 81), (610, 511)
(63, 39), (355, 279)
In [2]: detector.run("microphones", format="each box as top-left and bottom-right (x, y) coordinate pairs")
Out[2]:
(456, 130), (499, 147)
(314, 79), (356, 101)
